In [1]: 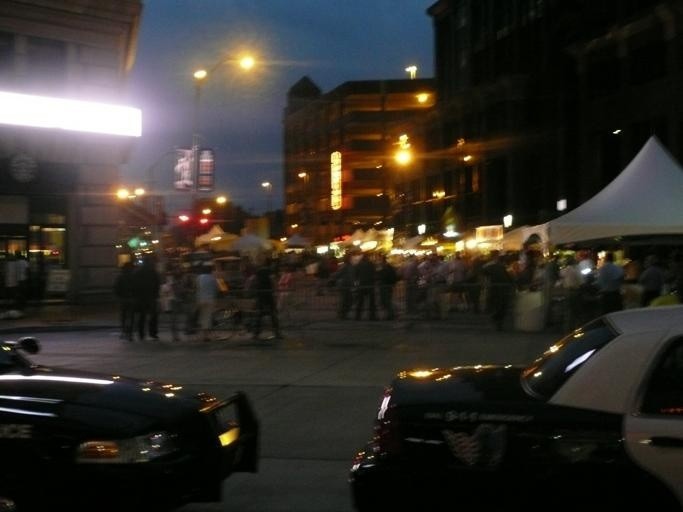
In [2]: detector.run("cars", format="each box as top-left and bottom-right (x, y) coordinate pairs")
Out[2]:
(156, 249), (247, 300)
(349, 303), (682, 512)
(0, 336), (263, 512)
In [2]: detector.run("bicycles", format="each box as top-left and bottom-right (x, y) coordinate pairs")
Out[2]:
(202, 294), (280, 343)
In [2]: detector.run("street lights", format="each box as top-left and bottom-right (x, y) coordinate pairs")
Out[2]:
(261, 178), (274, 214)
(381, 60), (419, 229)
(190, 50), (258, 244)
(298, 170), (314, 237)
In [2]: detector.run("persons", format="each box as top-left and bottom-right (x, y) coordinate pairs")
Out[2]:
(0, 234), (683, 345)
